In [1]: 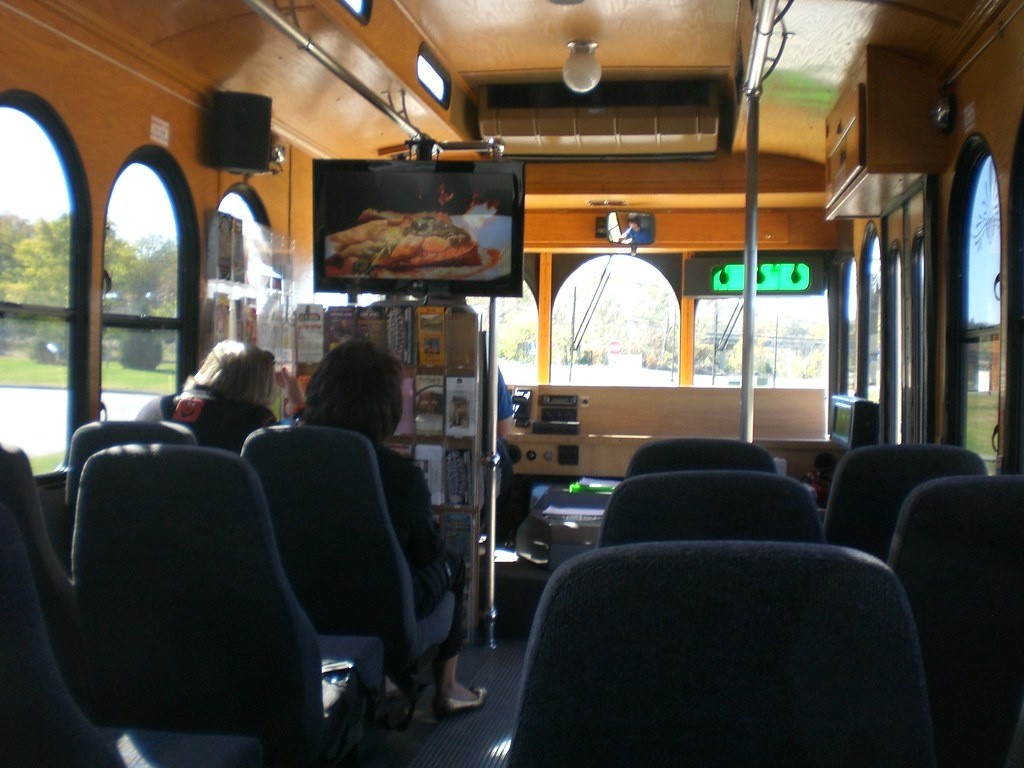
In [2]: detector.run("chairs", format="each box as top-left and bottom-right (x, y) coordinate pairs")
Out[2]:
(506, 437), (1024, 768)
(0, 393), (454, 768)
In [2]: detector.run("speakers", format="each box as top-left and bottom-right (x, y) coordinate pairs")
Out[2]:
(205, 91), (272, 174)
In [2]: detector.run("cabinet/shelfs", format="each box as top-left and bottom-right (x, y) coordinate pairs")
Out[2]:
(290, 311), (482, 647)
(825, 82), (944, 209)
(200, 210), (295, 423)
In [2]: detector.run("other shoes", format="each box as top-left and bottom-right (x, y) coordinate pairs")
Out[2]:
(432, 686), (488, 721)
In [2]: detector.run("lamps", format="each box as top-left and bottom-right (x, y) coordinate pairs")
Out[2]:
(562, 39), (602, 93)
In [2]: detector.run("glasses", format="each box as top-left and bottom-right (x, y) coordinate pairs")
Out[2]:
(262, 350), (276, 365)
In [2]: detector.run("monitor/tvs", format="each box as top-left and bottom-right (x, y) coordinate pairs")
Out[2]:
(828, 395), (878, 450)
(312, 159), (525, 299)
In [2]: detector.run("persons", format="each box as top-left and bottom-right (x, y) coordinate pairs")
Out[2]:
(619, 216), (651, 244)
(305, 337), (487, 722)
(496, 368), (514, 513)
(181, 338), (307, 427)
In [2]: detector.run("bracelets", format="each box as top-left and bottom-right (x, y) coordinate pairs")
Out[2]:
(296, 416), (304, 424)
